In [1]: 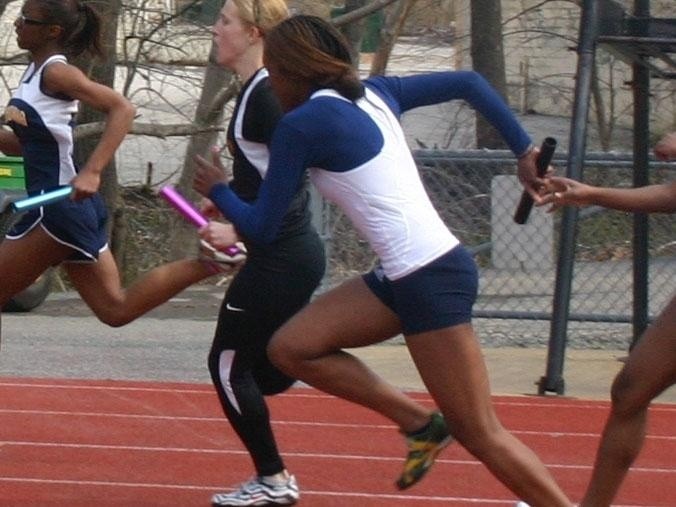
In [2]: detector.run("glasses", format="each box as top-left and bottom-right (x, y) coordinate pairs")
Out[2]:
(20, 9), (52, 26)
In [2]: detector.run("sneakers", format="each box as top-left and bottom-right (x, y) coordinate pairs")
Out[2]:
(395, 410), (455, 489)
(210, 474), (300, 507)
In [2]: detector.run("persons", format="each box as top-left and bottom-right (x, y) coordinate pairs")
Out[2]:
(206, 0), (327, 506)
(516, 128), (676, 505)
(0, 0), (247, 329)
(191, 14), (576, 507)
